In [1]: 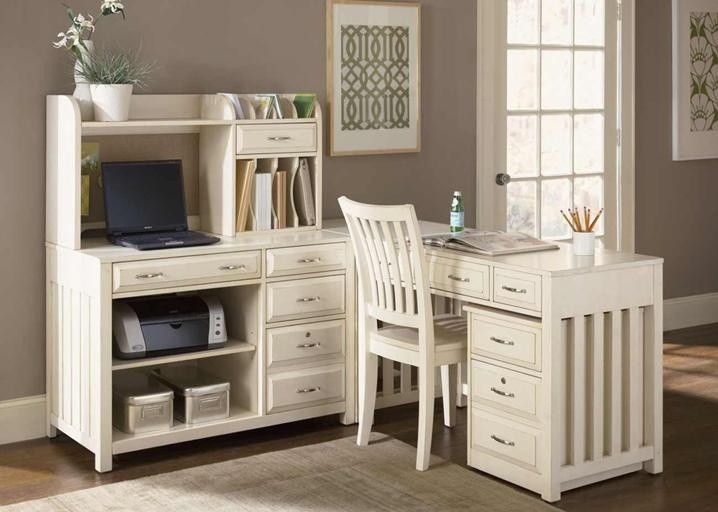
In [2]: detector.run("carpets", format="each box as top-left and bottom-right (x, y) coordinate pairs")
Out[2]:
(0, 432), (568, 512)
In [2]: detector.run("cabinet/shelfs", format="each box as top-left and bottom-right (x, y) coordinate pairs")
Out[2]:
(42, 91), (357, 473)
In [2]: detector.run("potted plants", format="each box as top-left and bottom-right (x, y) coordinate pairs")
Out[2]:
(76, 45), (160, 121)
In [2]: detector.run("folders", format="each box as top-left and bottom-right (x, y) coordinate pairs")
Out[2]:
(293, 158), (316, 226)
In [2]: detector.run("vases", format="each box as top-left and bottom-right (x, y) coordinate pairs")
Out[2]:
(70, 39), (96, 118)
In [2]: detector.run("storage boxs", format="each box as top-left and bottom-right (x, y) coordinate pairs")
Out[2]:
(151, 365), (231, 424)
(112, 371), (174, 434)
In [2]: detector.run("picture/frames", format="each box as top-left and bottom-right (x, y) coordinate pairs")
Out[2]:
(670, 0), (718, 162)
(324, 1), (424, 156)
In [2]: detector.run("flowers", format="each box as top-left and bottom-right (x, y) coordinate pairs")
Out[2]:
(47, 1), (126, 51)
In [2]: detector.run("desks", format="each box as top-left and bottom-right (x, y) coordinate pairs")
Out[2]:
(324, 215), (666, 504)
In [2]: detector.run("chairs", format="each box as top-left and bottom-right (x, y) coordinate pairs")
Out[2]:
(336, 193), (467, 472)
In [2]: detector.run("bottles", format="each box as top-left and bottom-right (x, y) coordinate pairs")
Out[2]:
(450, 190), (464, 232)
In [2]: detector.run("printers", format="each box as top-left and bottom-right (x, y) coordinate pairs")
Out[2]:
(112, 295), (228, 360)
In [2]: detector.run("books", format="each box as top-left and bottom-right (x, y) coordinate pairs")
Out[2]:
(393, 221), (559, 257)
(235, 157), (317, 231)
(216, 90), (318, 120)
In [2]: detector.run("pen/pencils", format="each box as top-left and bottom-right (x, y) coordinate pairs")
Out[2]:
(560, 206), (603, 232)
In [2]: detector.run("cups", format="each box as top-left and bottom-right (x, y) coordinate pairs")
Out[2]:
(572, 229), (594, 256)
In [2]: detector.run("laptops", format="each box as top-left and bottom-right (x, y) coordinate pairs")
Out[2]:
(102, 160), (221, 251)
